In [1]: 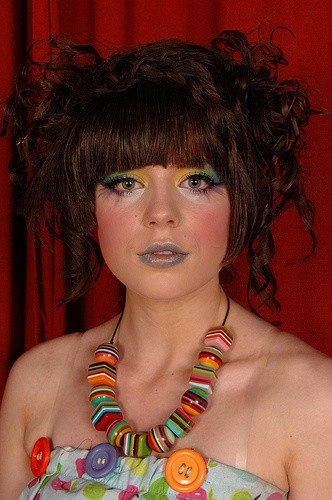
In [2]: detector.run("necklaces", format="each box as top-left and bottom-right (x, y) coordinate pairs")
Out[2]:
(87, 293), (236, 460)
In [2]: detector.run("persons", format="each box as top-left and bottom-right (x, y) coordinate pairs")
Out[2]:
(0, 20), (332, 500)
